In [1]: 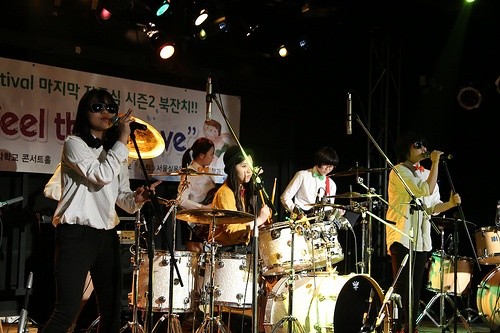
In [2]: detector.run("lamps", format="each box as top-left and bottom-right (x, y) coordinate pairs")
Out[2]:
(457, 86), (483, 110)
(86, 0), (309, 61)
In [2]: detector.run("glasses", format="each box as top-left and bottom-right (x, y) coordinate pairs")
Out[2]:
(415, 139), (428, 149)
(89, 102), (119, 113)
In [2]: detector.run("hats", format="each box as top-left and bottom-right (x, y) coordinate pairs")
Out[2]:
(223, 145), (254, 175)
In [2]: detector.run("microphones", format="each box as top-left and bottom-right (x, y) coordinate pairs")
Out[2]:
(205, 77), (212, 122)
(418, 151), (454, 160)
(111, 116), (147, 131)
(346, 91), (353, 134)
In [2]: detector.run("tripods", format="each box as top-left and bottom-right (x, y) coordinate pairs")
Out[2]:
(117, 201), (307, 333)
(416, 224), (473, 333)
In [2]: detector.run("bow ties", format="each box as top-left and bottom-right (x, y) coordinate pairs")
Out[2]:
(313, 172), (325, 181)
(415, 164), (424, 172)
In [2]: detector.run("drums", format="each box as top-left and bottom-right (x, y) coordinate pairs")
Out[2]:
(132, 247), (197, 313)
(197, 251), (260, 308)
(309, 221), (344, 268)
(425, 252), (474, 296)
(476, 265), (500, 333)
(264, 274), (394, 333)
(259, 221), (312, 275)
(475, 226), (500, 256)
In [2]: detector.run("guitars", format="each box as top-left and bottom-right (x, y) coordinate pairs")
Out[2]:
(290, 200), (382, 222)
(199, 165), (264, 206)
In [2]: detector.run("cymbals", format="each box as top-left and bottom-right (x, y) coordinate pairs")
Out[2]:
(176, 210), (254, 224)
(305, 203), (344, 208)
(324, 192), (381, 198)
(117, 113), (166, 159)
(433, 217), (476, 232)
(148, 169), (225, 176)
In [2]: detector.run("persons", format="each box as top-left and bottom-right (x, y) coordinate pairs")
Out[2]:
(39, 89), (166, 333)
(202, 119), (233, 177)
(386, 136), (461, 333)
(281, 148), (348, 223)
(175, 137), (221, 258)
(208, 144), (273, 253)
